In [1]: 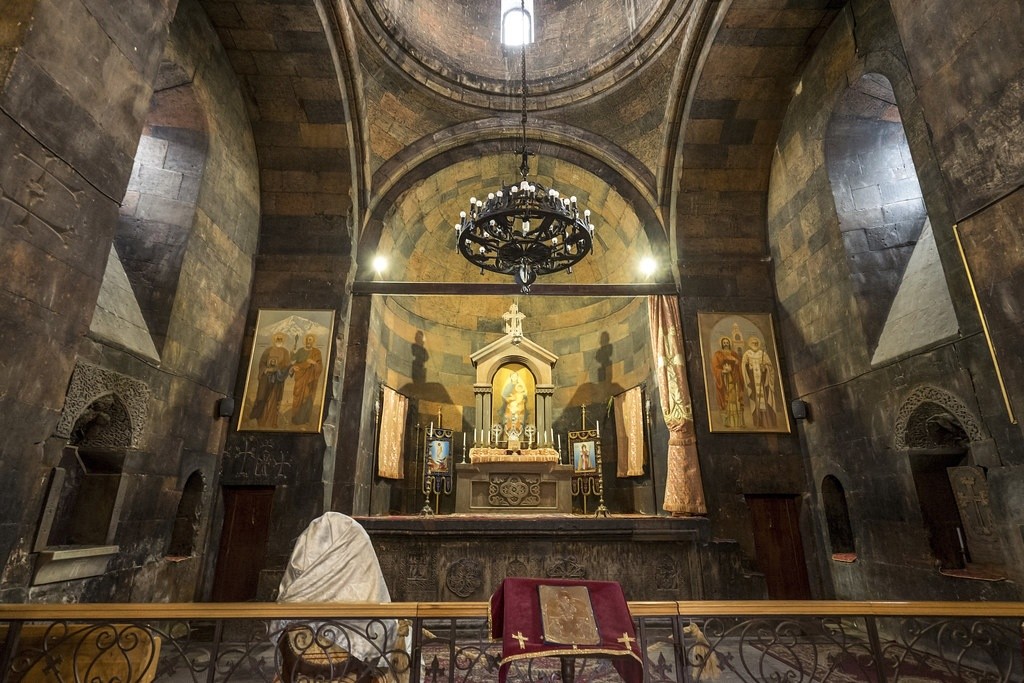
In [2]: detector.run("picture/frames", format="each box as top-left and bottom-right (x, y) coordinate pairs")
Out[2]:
(568, 429), (600, 477)
(235, 306), (336, 434)
(698, 311), (792, 435)
(424, 427), (453, 477)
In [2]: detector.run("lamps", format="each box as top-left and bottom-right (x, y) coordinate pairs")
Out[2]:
(453, 0), (596, 297)
